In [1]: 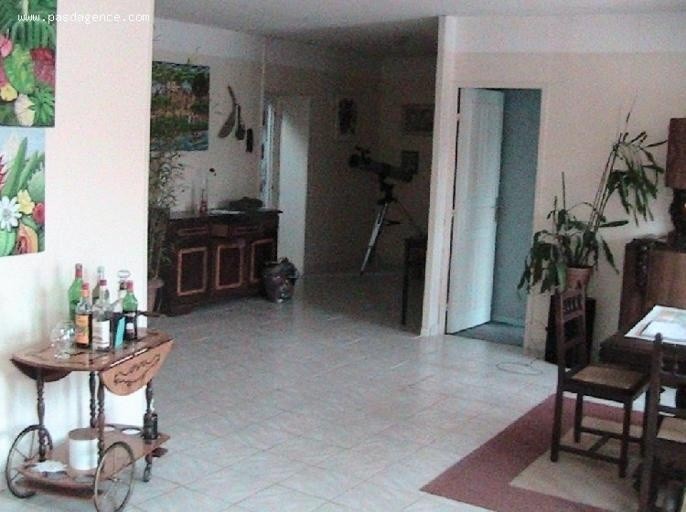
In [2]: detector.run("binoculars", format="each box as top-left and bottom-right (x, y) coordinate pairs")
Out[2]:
(348, 153), (412, 182)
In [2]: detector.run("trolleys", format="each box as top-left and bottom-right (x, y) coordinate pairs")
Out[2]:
(7, 311), (173, 512)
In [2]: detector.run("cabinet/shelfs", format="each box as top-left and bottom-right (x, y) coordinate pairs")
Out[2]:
(155, 208), (284, 317)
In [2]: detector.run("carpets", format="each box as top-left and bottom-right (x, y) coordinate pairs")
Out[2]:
(420, 393), (686, 512)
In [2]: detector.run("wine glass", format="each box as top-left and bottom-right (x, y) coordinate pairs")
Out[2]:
(51, 319), (76, 360)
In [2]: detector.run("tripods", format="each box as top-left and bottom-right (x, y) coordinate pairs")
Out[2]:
(360, 196), (420, 277)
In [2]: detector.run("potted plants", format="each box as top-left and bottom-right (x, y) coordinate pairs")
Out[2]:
(516, 90), (669, 296)
(148, 50), (196, 318)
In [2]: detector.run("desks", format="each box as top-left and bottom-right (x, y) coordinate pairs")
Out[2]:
(598, 302), (685, 479)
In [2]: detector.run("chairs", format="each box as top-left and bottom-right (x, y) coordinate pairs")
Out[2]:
(550, 287), (651, 478)
(639, 333), (686, 512)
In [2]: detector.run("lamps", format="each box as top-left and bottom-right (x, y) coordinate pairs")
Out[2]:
(666, 118), (686, 250)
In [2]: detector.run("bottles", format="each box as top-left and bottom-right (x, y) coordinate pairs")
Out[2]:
(68, 260), (137, 351)
